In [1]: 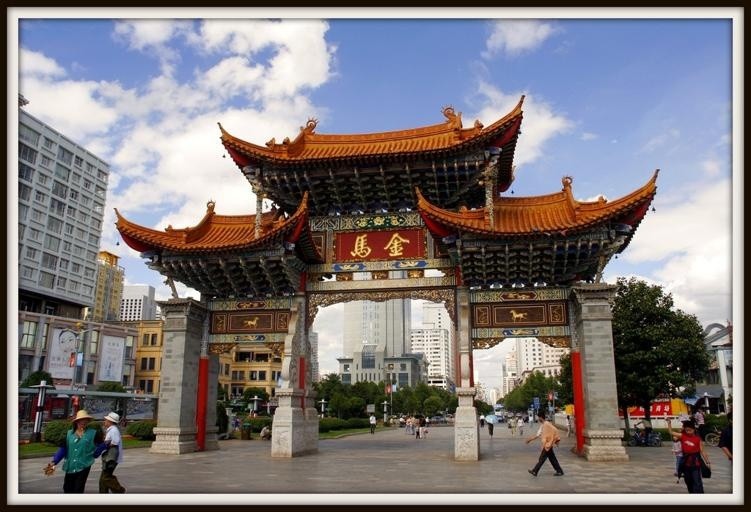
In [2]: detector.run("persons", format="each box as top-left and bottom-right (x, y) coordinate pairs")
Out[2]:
(259, 424), (271, 441)
(43, 409), (106, 493)
(387, 412), (432, 440)
(633, 418), (652, 446)
(98, 410), (126, 494)
(524, 411), (566, 476)
(230, 412), (242, 431)
(58, 329), (79, 360)
(565, 415), (575, 437)
(369, 413), (376, 435)
(478, 412), (526, 439)
(664, 407), (733, 493)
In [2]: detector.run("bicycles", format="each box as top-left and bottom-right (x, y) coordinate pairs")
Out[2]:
(704, 425), (722, 447)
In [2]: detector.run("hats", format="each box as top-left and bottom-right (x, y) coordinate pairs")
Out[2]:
(72, 410), (93, 423)
(103, 412), (120, 424)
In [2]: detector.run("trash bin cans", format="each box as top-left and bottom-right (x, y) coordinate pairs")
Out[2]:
(242, 422), (251, 440)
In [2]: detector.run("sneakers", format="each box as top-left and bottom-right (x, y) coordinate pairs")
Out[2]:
(528, 470), (536, 476)
(553, 473), (561, 476)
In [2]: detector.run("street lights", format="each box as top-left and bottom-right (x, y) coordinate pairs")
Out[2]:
(389, 363), (393, 421)
(61, 321), (104, 388)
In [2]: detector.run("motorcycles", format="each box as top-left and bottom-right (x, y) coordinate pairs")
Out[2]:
(626, 422), (662, 447)
